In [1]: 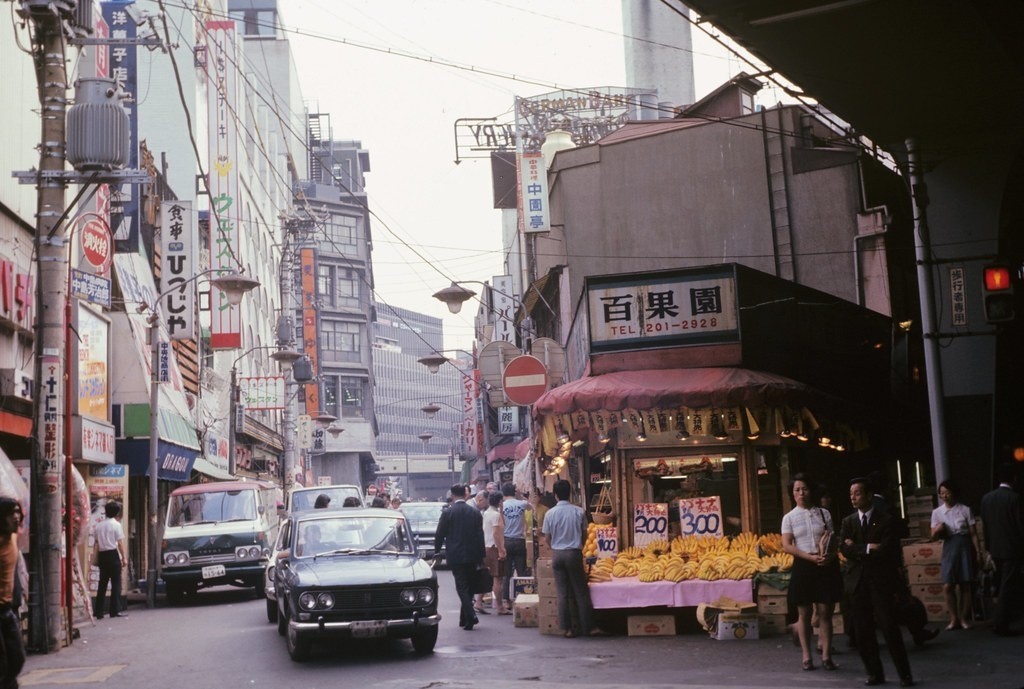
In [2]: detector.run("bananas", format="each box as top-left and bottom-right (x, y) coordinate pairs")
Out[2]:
(586, 532), (795, 583)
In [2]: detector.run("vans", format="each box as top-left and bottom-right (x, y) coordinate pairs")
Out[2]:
(161, 481), (279, 603)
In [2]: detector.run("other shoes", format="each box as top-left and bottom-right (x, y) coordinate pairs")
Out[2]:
(590, 628), (612, 638)
(565, 630), (576, 638)
(822, 657), (835, 670)
(460, 621), (465, 626)
(463, 615), (479, 630)
(991, 627), (1011, 635)
(802, 658), (813, 670)
(96, 615), (104, 620)
(110, 612), (124, 617)
(945, 621), (959, 631)
(959, 622), (971, 630)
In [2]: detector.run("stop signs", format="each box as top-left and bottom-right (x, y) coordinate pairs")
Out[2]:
(502, 355), (548, 407)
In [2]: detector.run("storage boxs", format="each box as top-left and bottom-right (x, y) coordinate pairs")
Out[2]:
(710, 582), (844, 640)
(628, 612), (677, 636)
(898, 488), (985, 623)
(509, 536), (567, 636)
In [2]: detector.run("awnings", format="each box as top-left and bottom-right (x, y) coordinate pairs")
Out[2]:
(191, 458), (238, 480)
(533, 366), (806, 429)
(487, 443), (519, 464)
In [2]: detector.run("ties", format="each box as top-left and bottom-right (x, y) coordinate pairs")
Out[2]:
(862, 514), (867, 534)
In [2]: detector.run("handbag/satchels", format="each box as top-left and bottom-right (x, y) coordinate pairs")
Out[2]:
(818, 508), (835, 557)
(471, 560), (494, 594)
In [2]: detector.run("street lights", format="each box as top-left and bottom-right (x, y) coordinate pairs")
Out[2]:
(433, 281), (538, 563)
(229, 344), (302, 477)
(146, 266), (261, 607)
(418, 430), (455, 486)
(417, 350), (493, 483)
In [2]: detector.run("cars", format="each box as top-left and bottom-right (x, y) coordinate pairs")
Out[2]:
(274, 508), (442, 662)
(399, 501), (450, 571)
(286, 485), (366, 513)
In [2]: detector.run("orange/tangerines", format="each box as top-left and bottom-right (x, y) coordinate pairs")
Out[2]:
(582, 523), (612, 557)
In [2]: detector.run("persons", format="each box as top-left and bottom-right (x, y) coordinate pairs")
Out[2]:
(315, 493), (401, 508)
(276, 525), (335, 559)
(541, 480), (610, 638)
(980, 463), (1024, 638)
(0, 496), (26, 689)
(435, 484), (486, 629)
(525, 489), (548, 528)
(92, 501), (128, 619)
(364, 526), (396, 550)
(929, 480), (981, 630)
(500, 482), (533, 609)
(781, 472), (840, 670)
(840, 477), (940, 685)
(473, 491), (513, 615)
(446, 479), (502, 513)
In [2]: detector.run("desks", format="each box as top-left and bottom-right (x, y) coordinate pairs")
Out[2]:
(588, 572), (754, 633)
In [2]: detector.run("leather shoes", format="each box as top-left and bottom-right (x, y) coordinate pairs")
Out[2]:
(900, 672), (912, 686)
(864, 676), (885, 686)
(913, 627), (940, 646)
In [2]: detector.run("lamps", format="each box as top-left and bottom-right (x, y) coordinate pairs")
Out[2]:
(538, 409), (847, 476)
